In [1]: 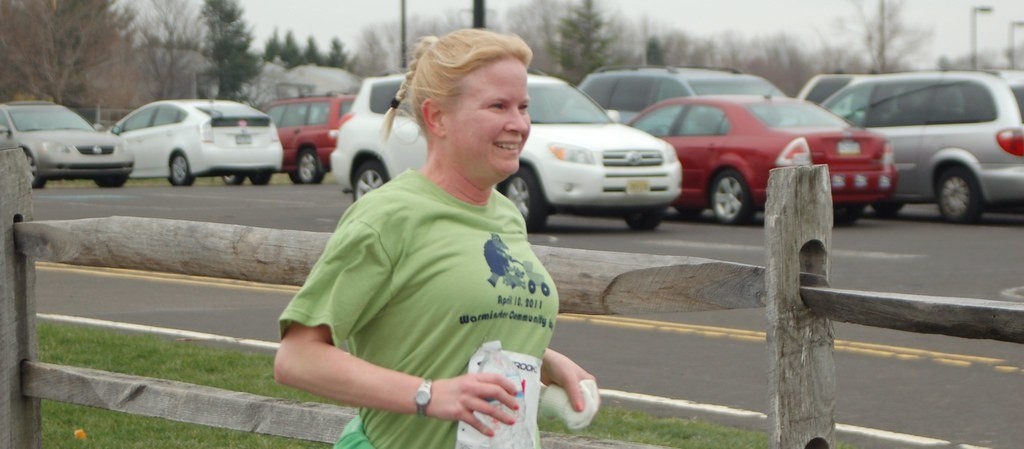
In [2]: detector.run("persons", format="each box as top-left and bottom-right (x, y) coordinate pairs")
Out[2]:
(273, 28), (595, 449)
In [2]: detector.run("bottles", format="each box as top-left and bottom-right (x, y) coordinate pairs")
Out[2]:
(475, 341), (540, 449)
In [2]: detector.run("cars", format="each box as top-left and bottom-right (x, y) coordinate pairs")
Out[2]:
(809, 68), (1024, 225)
(105, 98), (284, 187)
(328, 71), (683, 235)
(0, 100), (136, 189)
(248, 95), (355, 187)
(624, 93), (898, 228)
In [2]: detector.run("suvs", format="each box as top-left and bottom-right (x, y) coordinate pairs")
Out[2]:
(577, 63), (791, 135)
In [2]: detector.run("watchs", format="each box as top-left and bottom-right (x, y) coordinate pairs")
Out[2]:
(416, 378), (432, 416)
